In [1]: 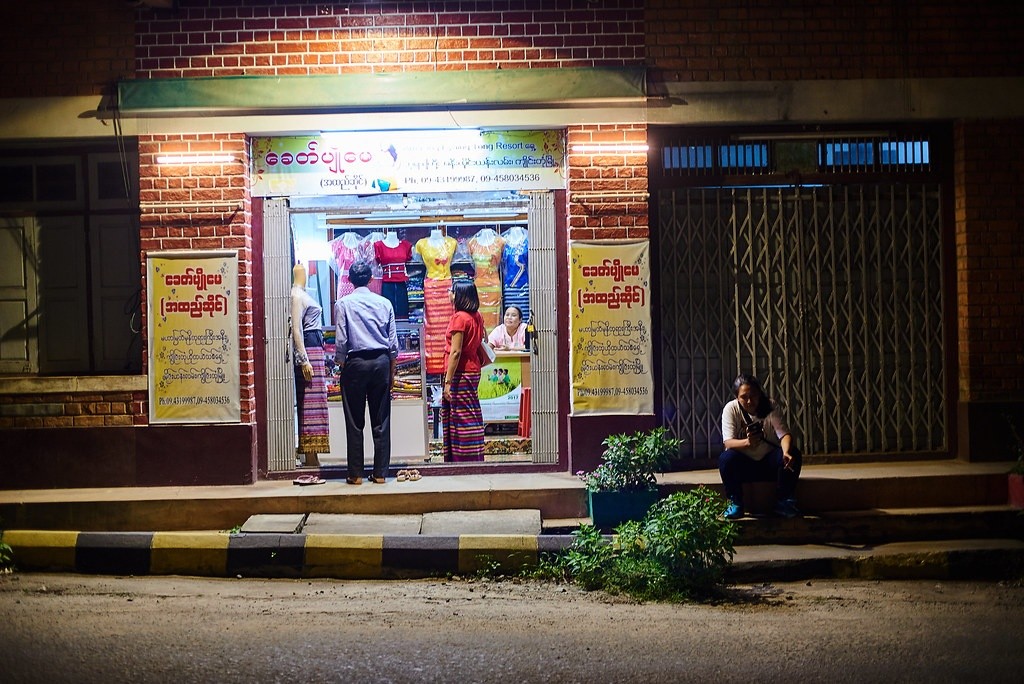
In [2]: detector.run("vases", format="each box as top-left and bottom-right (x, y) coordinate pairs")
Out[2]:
(589, 490), (658, 535)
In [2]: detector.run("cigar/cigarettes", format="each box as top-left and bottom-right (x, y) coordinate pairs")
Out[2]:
(785, 463), (794, 472)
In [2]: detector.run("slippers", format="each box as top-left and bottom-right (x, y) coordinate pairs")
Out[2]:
(293, 474), (327, 485)
(397, 469), (407, 482)
(409, 469), (422, 480)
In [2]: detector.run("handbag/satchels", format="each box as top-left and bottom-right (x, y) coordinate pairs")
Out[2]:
(476, 327), (496, 367)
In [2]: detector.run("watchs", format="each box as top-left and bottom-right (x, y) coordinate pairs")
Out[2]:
(445, 379), (451, 384)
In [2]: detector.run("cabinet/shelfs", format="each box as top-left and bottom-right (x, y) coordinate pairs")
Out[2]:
(395, 258), (477, 322)
(477, 351), (532, 422)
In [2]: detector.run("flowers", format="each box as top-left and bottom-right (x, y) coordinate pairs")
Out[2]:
(576, 426), (684, 490)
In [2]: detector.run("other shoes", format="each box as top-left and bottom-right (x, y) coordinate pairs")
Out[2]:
(346, 476), (362, 484)
(367, 475), (385, 483)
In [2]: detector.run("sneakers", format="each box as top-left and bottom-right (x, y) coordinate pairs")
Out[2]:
(722, 499), (744, 519)
(774, 500), (797, 517)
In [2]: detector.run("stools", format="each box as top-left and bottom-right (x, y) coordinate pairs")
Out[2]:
(517, 387), (531, 438)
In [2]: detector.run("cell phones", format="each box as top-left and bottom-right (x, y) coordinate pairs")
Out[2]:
(748, 422), (761, 436)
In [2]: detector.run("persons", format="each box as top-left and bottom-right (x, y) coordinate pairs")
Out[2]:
(415, 225), (458, 374)
(718, 375), (802, 519)
(467, 227), (507, 334)
(330, 233), (413, 319)
(501, 227), (529, 326)
(292, 264), (330, 466)
(335, 261), (398, 485)
(442, 281), (488, 463)
(487, 305), (528, 351)
